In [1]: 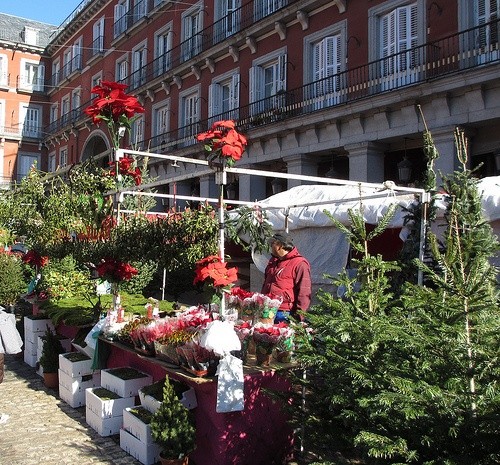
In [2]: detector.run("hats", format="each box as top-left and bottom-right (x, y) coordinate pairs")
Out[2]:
(272, 231), (294, 246)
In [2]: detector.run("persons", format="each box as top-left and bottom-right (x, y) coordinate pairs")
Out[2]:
(261, 231), (311, 324)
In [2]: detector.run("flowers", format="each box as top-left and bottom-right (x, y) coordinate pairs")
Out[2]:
(23, 76), (315, 376)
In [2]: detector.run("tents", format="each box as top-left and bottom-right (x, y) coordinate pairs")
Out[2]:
(229, 176), (500, 314)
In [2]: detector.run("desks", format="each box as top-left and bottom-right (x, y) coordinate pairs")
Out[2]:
(99, 336), (302, 465)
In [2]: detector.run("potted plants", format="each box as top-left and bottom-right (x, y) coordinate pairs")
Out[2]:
(24, 315), (198, 465)
